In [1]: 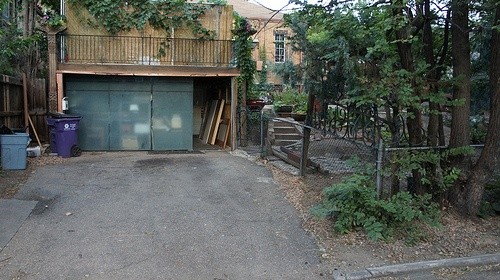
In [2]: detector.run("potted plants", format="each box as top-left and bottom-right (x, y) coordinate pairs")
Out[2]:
(247, 84), (309, 122)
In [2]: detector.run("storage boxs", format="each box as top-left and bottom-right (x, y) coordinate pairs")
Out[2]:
(0, 133), (30, 170)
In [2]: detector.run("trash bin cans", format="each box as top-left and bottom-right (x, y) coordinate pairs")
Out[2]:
(46, 111), (83, 159)
(0, 124), (30, 171)
